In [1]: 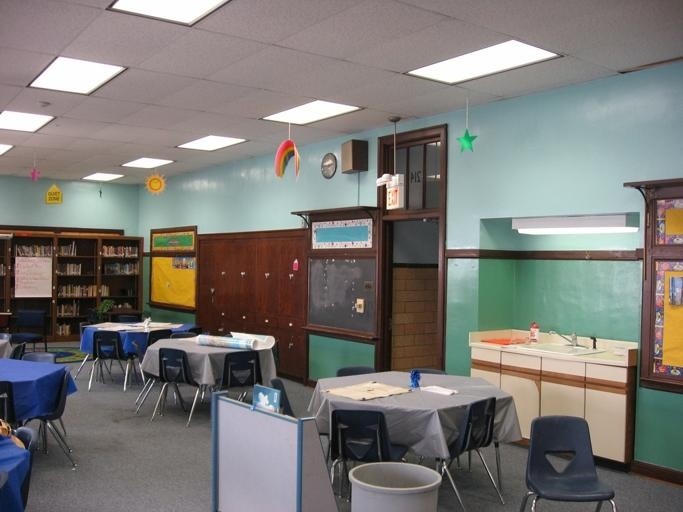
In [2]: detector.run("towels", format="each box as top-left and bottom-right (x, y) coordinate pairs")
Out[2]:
(481, 337), (525, 345)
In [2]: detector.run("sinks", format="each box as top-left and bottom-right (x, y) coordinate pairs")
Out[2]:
(514, 342), (606, 357)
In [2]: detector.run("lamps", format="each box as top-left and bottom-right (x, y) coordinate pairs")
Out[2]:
(511, 212), (638, 233)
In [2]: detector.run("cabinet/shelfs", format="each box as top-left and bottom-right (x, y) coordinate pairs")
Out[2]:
(0, 229), (54, 342)
(470, 343), (541, 448)
(54, 230), (143, 342)
(206, 387), (338, 512)
(541, 355), (634, 472)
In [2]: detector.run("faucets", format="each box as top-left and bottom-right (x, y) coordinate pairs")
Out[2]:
(547, 329), (578, 347)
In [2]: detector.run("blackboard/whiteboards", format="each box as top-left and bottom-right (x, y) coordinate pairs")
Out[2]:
(14, 256), (53, 298)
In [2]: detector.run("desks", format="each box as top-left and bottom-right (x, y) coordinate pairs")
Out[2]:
(304, 370), (521, 497)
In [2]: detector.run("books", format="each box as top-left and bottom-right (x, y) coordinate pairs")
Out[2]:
(16, 240), (138, 337)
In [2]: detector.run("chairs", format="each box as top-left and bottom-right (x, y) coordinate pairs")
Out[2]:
(417, 394), (506, 511)
(328, 408), (408, 503)
(337, 366), (381, 376)
(272, 378), (332, 467)
(0, 310), (79, 512)
(406, 369), (464, 470)
(517, 415), (616, 511)
(74, 321), (276, 429)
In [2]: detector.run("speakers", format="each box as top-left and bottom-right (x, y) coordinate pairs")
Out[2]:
(341, 138), (367, 174)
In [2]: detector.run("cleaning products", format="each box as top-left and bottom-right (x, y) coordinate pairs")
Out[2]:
(530, 321), (539, 343)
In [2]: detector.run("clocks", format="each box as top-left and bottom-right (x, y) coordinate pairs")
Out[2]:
(320, 153), (336, 178)
